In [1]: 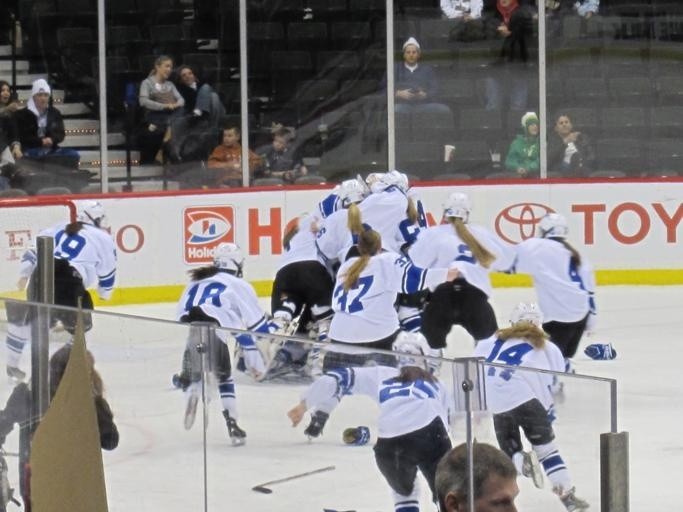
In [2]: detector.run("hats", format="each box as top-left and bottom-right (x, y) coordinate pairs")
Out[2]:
(31, 77), (53, 99)
(521, 112), (539, 138)
(403, 36), (422, 53)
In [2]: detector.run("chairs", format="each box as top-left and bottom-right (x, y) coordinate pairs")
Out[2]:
(0, 0), (683, 198)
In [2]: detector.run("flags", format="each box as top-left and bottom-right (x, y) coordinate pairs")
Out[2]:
(28, 297), (109, 512)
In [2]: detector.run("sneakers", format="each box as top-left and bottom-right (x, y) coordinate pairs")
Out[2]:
(553, 486), (590, 512)
(222, 408), (246, 446)
(263, 348), (295, 379)
(5, 366), (25, 380)
(521, 450), (543, 489)
(185, 395), (197, 429)
(304, 411), (329, 442)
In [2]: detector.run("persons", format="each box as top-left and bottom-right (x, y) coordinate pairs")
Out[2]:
(3, 170), (617, 512)
(1, 2), (601, 177)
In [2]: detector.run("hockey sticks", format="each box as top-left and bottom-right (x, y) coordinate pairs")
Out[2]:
(251, 467), (335, 494)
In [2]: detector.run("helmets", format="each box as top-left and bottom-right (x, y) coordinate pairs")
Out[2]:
(211, 241), (250, 274)
(393, 334), (431, 370)
(337, 171), (570, 242)
(74, 200), (108, 230)
